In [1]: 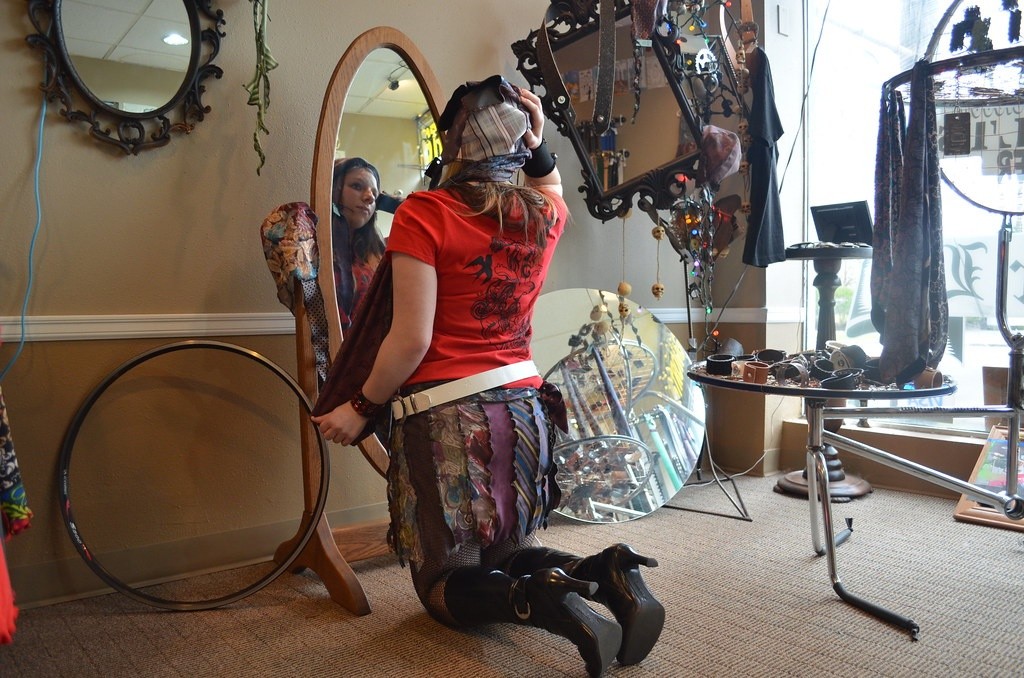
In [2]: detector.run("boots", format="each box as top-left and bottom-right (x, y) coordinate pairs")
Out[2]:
(510, 543), (667, 666)
(445, 566), (623, 675)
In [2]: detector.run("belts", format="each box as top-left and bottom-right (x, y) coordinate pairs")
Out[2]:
(391, 358), (539, 425)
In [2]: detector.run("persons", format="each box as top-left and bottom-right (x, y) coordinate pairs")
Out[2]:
(330, 156), (407, 339)
(310, 74), (667, 678)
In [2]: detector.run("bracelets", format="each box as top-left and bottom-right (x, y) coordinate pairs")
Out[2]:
(707, 340), (943, 390)
(350, 390), (379, 417)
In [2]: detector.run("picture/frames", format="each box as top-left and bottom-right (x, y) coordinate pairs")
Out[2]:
(953, 424), (1024, 532)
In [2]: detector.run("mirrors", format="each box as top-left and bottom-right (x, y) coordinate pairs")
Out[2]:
(510, 0), (721, 223)
(24, 0), (226, 155)
(274, 32), (447, 617)
